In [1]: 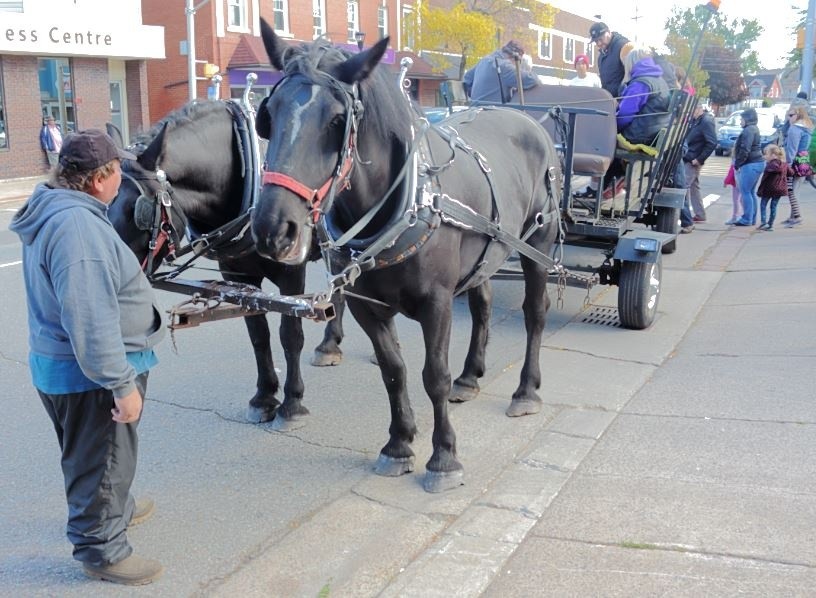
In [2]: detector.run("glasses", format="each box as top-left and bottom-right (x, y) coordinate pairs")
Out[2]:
(789, 113), (797, 117)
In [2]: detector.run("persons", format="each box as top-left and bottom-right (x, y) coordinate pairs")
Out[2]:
(572, 21), (639, 199)
(463, 40), (539, 110)
(40, 115), (64, 174)
(672, 137), (695, 233)
(781, 92), (807, 139)
(568, 54), (603, 87)
(602, 41), (680, 200)
(785, 105), (814, 228)
(733, 109), (765, 226)
(9, 128), (163, 587)
(756, 144), (795, 231)
(723, 146), (745, 225)
(680, 100), (717, 223)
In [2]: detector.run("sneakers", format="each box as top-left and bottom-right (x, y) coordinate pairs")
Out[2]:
(681, 225), (695, 234)
(692, 215), (706, 223)
(82, 555), (164, 586)
(603, 175), (624, 200)
(126, 496), (155, 526)
(573, 186), (603, 203)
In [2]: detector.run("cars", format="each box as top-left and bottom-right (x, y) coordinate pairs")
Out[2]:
(716, 108), (778, 156)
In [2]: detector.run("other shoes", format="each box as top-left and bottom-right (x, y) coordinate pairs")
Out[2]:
(781, 216), (794, 224)
(765, 226), (775, 231)
(785, 218), (805, 227)
(725, 217), (739, 224)
(735, 221), (744, 226)
(757, 223), (770, 230)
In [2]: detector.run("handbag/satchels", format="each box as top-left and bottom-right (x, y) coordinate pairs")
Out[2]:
(792, 151), (816, 177)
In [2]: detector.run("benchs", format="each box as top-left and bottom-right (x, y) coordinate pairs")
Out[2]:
(437, 80), (472, 108)
(506, 83), (617, 223)
(588, 88), (700, 219)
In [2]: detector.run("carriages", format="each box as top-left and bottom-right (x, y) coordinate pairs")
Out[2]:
(107, 15), (696, 492)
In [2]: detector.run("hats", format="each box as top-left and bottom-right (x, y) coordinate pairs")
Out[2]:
(507, 40), (524, 64)
(587, 22), (610, 44)
(574, 54), (589, 69)
(58, 129), (138, 171)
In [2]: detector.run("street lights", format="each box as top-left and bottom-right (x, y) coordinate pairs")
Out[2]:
(353, 31), (365, 54)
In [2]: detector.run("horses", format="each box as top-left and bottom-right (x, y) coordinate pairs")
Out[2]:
(106, 96), (346, 433)
(252, 16), (561, 493)
